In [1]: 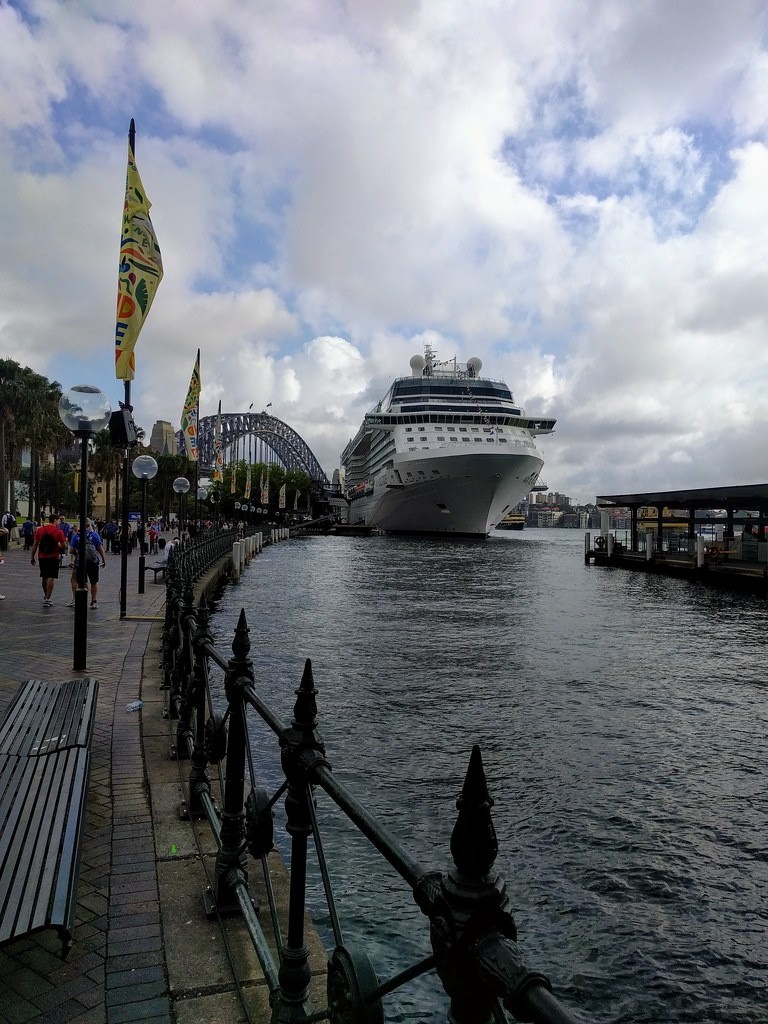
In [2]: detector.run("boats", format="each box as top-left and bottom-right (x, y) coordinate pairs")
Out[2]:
(634, 506), (689, 544)
(495, 513), (526, 531)
(698, 525), (725, 534)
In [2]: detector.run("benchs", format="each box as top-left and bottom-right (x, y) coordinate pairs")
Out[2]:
(0, 677), (101, 962)
(144, 560), (167, 585)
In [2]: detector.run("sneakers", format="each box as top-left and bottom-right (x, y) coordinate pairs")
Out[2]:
(90, 600), (98, 609)
(42, 596), (53, 607)
(65, 600), (75, 608)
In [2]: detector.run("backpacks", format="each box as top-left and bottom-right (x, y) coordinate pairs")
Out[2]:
(77, 532), (99, 565)
(38, 526), (60, 554)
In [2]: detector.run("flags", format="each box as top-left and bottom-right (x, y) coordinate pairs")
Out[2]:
(264, 473), (270, 505)
(231, 448), (237, 495)
(293, 489), (302, 511)
(246, 460), (252, 501)
(260, 474), (265, 504)
(180, 358), (201, 463)
(113, 134), (164, 384)
(278, 483), (286, 509)
(213, 411), (225, 484)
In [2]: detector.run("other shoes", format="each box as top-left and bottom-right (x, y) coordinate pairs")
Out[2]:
(23, 547), (29, 551)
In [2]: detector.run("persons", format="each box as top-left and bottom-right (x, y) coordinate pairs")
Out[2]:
(41, 508), (47, 527)
(67, 517), (106, 609)
(57, 515), (71, 554)
(2, 510), (16, 542)
(68, 523), (80, 568)
(30, 514), (68, 607)
(16, 507), (21, 516)
(91, 516), (249, 563)
(21, 517), (34, 550)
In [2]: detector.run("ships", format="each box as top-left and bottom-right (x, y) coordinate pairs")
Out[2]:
(338, 343), (557, 546)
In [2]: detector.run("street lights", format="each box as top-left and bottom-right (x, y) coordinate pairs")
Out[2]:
(169, 476), (306, 549)
(57, 384), (112, 672)
(130, 455), (159, 594)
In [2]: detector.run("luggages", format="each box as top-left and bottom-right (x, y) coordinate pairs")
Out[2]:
(144, 542), (149, 555)
(127, 542), (132, 555)
(153, 535), (159, 555)
(112, 535), (121, 555)
(58, 540), (71, 568)
